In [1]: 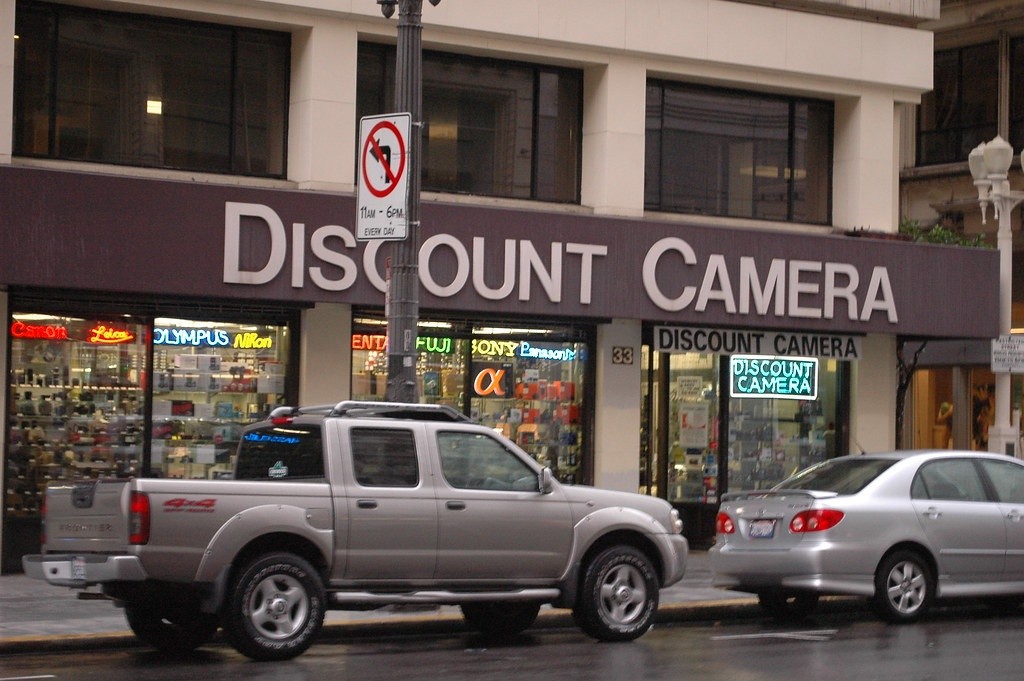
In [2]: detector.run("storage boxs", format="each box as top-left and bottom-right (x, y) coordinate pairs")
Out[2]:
(257, 363), (285, 393)
(153, 399), (233, 419)
(153, 354), (221, 391)
(351, 373), (388, 395)
(152, 426), (239, 477)
(221, 362), (249, 370)
(510, 378), (580, 423)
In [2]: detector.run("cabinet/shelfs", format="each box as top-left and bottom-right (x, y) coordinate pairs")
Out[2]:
(729, 418), (827, 463)
(421, 395), (582, 474)
(5, 369), (282, 518)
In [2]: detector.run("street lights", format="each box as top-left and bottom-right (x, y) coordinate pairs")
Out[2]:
(967, 133), (1024, 458)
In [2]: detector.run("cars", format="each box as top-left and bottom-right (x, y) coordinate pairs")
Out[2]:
(709, 449), (1024, 622)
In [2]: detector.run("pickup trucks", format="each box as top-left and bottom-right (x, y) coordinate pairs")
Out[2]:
(20, 399), (688, 661)
